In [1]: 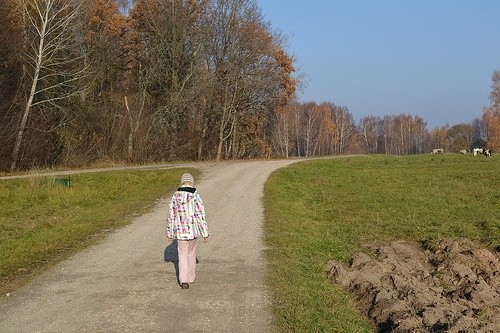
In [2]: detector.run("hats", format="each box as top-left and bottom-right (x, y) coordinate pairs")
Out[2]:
(181, 173), (194, 184)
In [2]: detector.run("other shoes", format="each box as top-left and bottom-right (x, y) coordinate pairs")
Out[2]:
(182, 283), (189, 289)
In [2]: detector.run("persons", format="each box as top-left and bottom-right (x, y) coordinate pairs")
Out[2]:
(166, 173), (209, 289)
